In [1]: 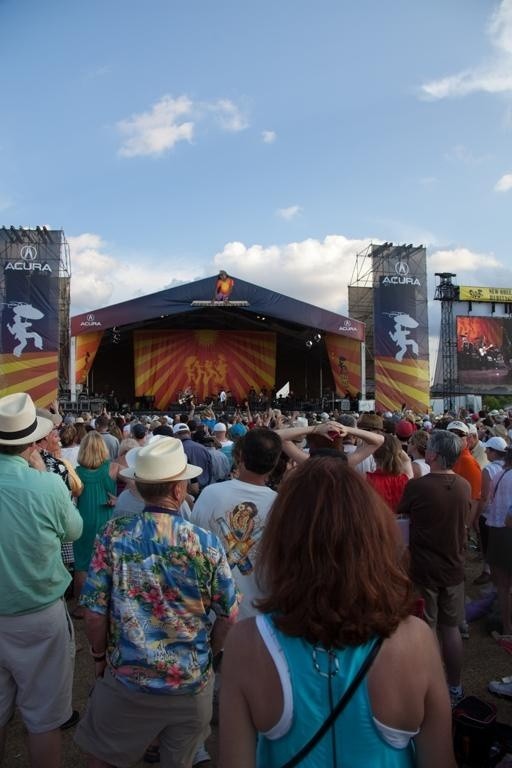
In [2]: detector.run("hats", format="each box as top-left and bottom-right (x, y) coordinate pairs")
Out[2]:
(280, 421), (307, 448)
(415, 417), (421, 420)
(470, 415), (479, 422)
(488, 409), (499, 416)
(486, 424), (510, 445)
(214, 422), (226, 432)
(406, 414), (416, 422)
(485, 437), (508, 453)
(447, 421), (469, 435)
(467, 423), (478, 434)
(173, 423), (190, 434)
(396, 420), (417, 437)
(384, 412), (392, 419)
(118, 435), (203, 483)
(0, 392), (52, 446)
(36, 407), (63, 429)
(416, 420), (423, 427)
(424, 421), (433, 429)
(319, 412), (329, 419)
(229, 424), (247, 439)
(499, 409), (504, 415)
(357, 414), (385, 431)
(76, 417), (85, 423)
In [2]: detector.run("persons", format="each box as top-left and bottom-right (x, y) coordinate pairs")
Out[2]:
(226, 401), (359, 428)
(336, 415), (376, 474)
(73, 417), (90, 447)
(407, 432), (430, 477)
(400, 430), (472, 698)
(447, 421), (481, 639)
(217, 386), (226, 407)
(95, 416), (119, 459)
(262, 386), (267, 396)
(275, 422), (383, 469)
(188, 398), (217, 429)
(487, 506), (512, 699)
(191, 427), (283, 626)
(365, 433), (409, 515)
(214, 423), (232, 446)
(220, 424), (246, 467)
(74, 435), (239, 768)
(37, 413), (71, 492)
(402, 408), (512, 427)
(220, 456), (454, 767)
(132, 424), (146, 444)
(487, 446), (512, 643)
(173, 423), (214, 489)
(184, 385), (193, 398)
(479, 336), (491, 369)
(59, 425), (84, 487)
(74, 430), (134, 619)
(36, 408), (83, 563)
(81, 412), (95, 432)
(114, 412), (187, 429)
(249, 385), (256, 398)
(472, 436), (507, 556)
(466, 423), (489, 469)
(0, 393), (83, 768)
(272, 385), (277, 396)
(193, 431), (230, 481)
(396, 421), (412, 451)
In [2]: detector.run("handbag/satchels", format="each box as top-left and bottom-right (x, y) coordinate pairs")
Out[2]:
(452, 696), (512, 768)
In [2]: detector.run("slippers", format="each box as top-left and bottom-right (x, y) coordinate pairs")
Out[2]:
(70, 606), (84, 619)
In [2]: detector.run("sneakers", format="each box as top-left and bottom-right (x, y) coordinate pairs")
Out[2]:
(144, 745), (161, 763)
(486, 676), (512, 700)
(461, 624), (471, 640)
(61, 711), (80, 730)
(472, 571), (493, 586)
(449, 685), (466, 711)
(193, 743), (211, 766)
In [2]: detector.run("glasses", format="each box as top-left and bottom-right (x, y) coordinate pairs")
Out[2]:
(416, 420), (420, 422)
(451, 431), (466, 437)
